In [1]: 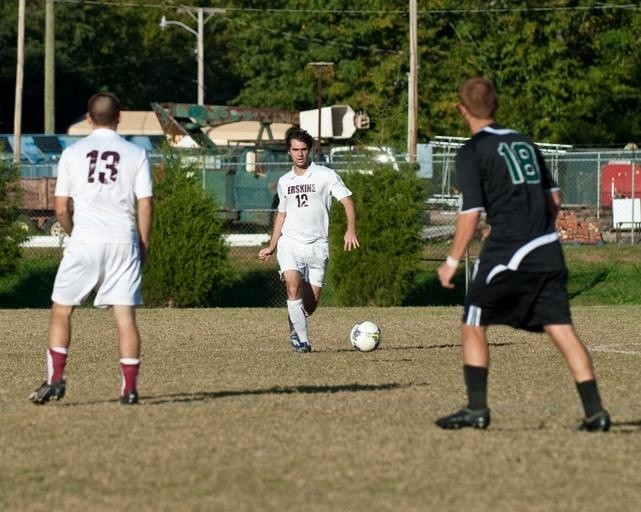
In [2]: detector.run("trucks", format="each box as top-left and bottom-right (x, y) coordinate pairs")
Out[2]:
(0, 100), (372, 227)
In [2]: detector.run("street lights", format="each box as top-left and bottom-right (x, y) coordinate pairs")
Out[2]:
(159, 7), (206, 105)
(306, 60), (334, 157)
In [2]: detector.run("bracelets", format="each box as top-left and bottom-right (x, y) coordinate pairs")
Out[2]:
(446, 256), (461, 268)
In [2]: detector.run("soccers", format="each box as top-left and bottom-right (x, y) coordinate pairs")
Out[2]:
(349, 321), (381, 352)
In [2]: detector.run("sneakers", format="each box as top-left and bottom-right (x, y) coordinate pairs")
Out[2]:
(437, 408), (490, 428)
(289, 331), (311, 352)
(578, 411), (609, 432)
(121, 390), (138, 405)
(30, 380), (65, 405)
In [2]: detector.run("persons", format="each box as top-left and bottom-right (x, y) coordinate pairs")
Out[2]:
(435, 77), (611, 434)
(29, 93), (156, 404)
(257, 127), (360, 353)
